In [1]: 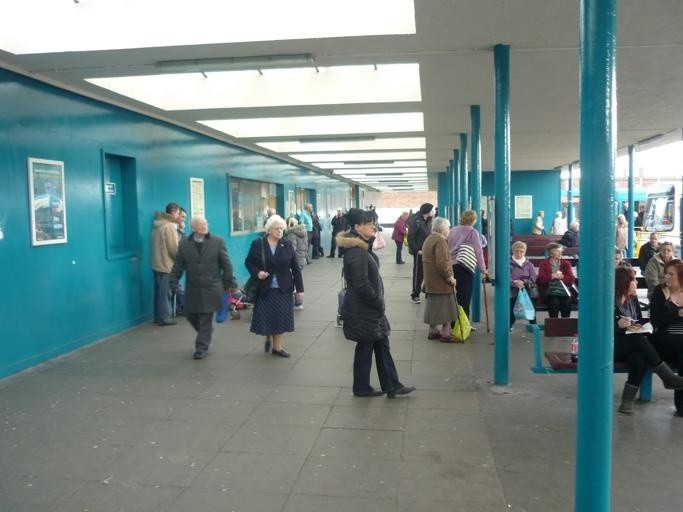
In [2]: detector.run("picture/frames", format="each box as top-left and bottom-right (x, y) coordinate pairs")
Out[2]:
(26, 157), (68, 247)
(189, 176), (205, 220)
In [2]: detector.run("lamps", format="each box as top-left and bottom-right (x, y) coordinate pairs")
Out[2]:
(151, 52), (319, 74)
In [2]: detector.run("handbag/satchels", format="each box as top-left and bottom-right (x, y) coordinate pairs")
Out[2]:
(244, 275), (261, 298)
(546, 280), (571, 298)
(455, 244), (477, 273)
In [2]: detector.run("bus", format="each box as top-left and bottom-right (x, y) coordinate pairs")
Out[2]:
(638, 181), (682, 264)
(559, 188), (645, 232)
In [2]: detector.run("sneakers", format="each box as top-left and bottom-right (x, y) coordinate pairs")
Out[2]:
(427, 331), (441, 340)
(319, 248), (324, 257)
(327, 256), (335, 257)
(441, 335), (461, 343)
(411, 297), (421, 303)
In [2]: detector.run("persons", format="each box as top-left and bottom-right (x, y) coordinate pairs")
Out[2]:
(244, 215), (304, 357)
(264, 208), (276, 227)
(287, 203), (489, 400)
(176, 207), (187, 296)
(151, 202), (178, 324)
(510, 200), (682, 416)
(169, 213), (234, 359)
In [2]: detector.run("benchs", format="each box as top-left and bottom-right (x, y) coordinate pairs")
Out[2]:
(524, 317), (682, 404)
(497, 258), (649, 325)
(509, 233), (578, 257)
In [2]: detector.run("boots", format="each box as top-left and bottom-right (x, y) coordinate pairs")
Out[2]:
(620, 381), (637, 413)
(654, 361), (683, 390)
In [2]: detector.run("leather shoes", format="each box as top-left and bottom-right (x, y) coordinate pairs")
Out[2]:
(194, 352), (206, 358)
(273, 348), (290, 358)
(153, 317), (176, 326)
(265, 340), (271, 352)
(369, 389), (382, 396)
(387, 386), (416, 398)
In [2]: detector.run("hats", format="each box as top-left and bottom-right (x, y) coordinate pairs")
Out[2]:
(421, 203), (433, 214)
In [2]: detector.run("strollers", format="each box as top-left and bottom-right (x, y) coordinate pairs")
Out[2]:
(230, 289), (253, 319)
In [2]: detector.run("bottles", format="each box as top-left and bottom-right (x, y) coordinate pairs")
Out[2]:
(569, 333), (578, 362)
